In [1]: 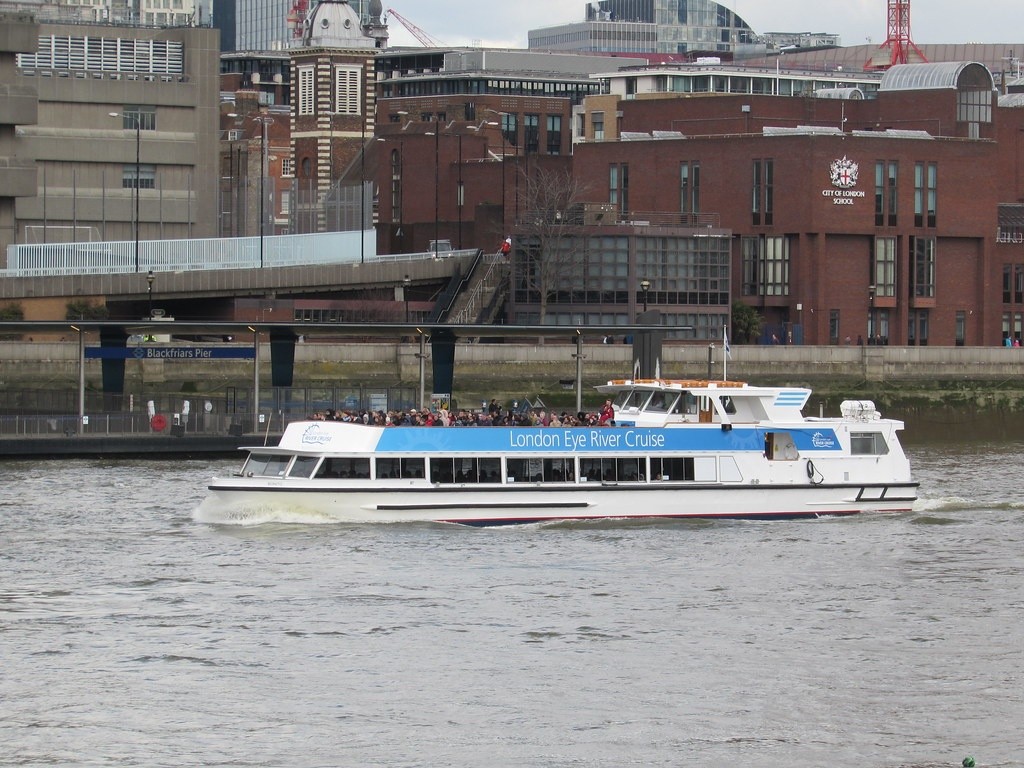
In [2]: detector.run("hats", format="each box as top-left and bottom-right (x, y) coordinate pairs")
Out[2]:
(411, 409), (416, 412)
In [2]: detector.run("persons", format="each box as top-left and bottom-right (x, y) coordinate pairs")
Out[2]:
(501, 235), (512, 261)
(324, 468), (661, 483)
(908, 334), (915, 345)
(313, 398), (616, 427)
(1002, 336), (1024, 347)
(772, 334), (780, 345)
(845, 334), (888, 345)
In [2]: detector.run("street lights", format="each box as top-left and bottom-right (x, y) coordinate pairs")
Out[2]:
(107, 111), (140, 272)
(397, 110), (439, 259)
(487, 121), (530, 225)
(465, 125), (505, 240)
(424, 131), (462, 251)
(868, 282), (876, 346)
(226, 112), (265, 269)
(403, 274), (413, 343)
(639, 277), (650, 313)
(145, 271), (155, 342)
(377, 138), (403, 254)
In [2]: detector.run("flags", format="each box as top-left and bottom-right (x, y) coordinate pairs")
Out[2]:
(726, 335), (732, 359)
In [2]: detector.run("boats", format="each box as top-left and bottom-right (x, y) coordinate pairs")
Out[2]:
(195, 323), (920, 527)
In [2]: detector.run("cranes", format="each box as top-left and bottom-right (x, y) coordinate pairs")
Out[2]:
(383, 8), (451, 47)
